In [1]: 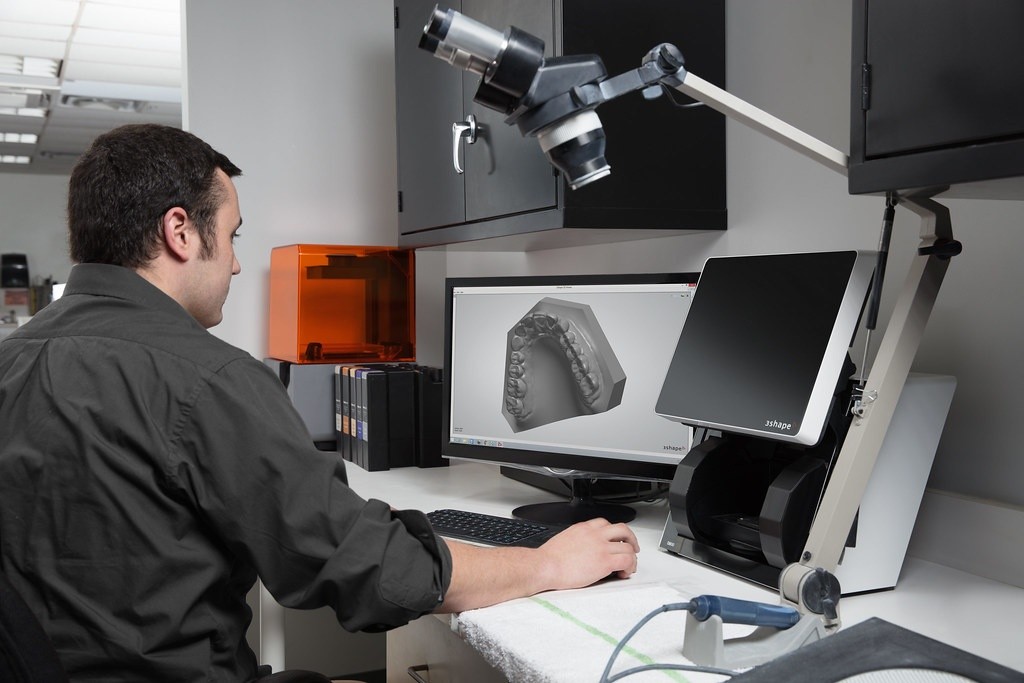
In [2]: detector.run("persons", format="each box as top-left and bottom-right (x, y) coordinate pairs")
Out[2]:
(0, 122), (641, 683)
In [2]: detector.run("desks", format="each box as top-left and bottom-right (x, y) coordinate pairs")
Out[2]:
(259, 459), (1024, 683)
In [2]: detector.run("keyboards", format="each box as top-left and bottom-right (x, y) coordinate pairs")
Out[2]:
(426, 509), (569, 547)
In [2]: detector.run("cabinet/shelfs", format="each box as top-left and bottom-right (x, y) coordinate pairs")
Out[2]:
(848, 0), (1024, 197)
(393, 0), (727, 251)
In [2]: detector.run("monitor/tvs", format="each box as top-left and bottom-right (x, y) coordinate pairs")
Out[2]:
(441, 272), (701, 530)
(655, 248), (879, 446)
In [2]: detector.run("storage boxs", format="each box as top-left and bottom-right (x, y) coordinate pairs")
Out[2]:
(271, 244), (417, 364)
(264, 358), (337, 450)
